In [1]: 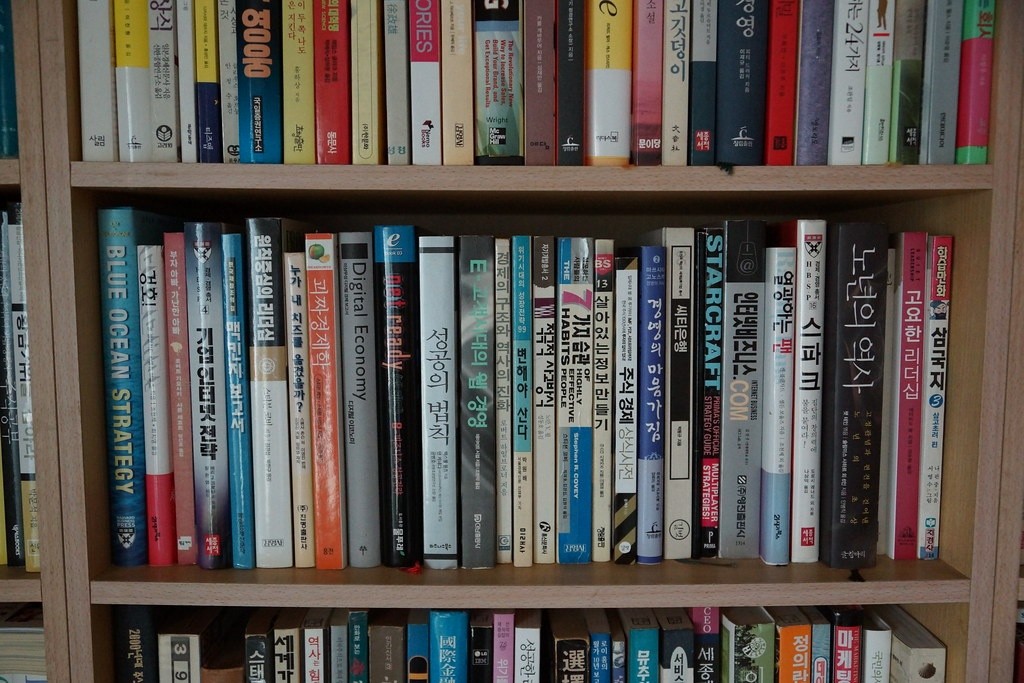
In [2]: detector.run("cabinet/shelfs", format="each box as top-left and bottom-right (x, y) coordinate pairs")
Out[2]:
(0, 0), (1024, 683)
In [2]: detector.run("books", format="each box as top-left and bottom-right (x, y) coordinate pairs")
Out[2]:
(1, 0), (993, 165)
(1, 606), (948, 683)
(0, 203), (956, 581)
